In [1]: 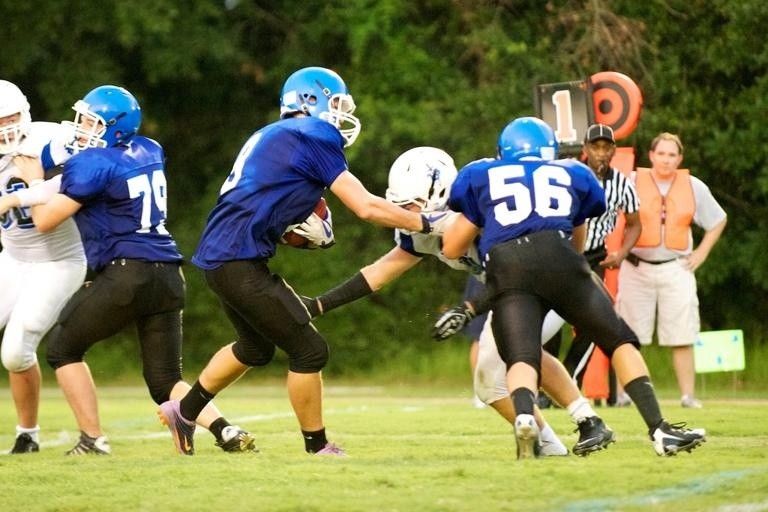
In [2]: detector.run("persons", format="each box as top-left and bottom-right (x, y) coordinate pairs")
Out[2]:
(0, 78), (88, 454)
(442, 116), (708, 461)
(12, 84), (256, 456)
(615, 132), (728, 408)
(535, 123), (643, 409)
(155, 66), (462, 459)
(299, 145), (616, 458)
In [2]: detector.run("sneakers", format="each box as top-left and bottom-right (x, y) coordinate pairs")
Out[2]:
(306, 443), (346, 457)
(650, 419), (706, 457)
(218, 426), (259, 454)
(11, 433), (39, 455)
(514, 415), (615, 459)
(157, 399), (195, 455)
(66, 432), (111, 455)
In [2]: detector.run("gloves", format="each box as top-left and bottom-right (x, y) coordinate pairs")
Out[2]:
(430, 302), (474, 342)
(419, 212), (458, 237)
(293, 206), (336, 249)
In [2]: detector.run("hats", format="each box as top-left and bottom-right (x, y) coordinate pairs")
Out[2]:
(584, 124), (615, 145)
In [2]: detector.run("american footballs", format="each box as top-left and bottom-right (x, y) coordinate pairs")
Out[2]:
(282, 196), (327, 247)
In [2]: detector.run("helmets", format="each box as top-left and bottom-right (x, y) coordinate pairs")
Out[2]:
(280, 66), (360, 148)
(386, 146), (458, 211)
(0, 81), (32, 155)
(62, 85), (140, 155)
(496, 117), (559, 162)
